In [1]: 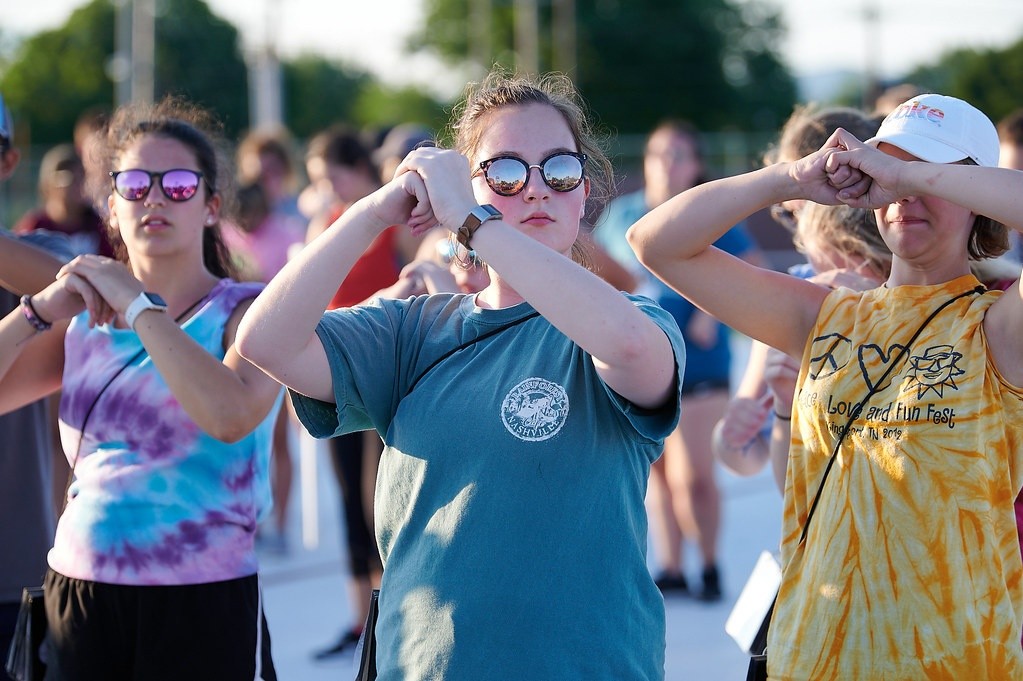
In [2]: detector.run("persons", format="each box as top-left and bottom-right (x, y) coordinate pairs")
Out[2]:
(628, 92), (1023, 681)
(0, 109), (640, 681)
(234, 68), (687, 681)
(0, 101), (288, 681)
(592, 118), (774, 604)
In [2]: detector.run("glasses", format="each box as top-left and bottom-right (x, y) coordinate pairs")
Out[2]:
(108, 168), (204, 202)
(469, 152), (588, 193)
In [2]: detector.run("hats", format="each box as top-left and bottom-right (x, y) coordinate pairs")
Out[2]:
(861, 94), (1000, 168)
(368, 122), (438, 168)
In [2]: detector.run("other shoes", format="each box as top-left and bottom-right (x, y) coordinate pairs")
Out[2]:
(700, 571), (721, 601)
(649, 574), (686, 590)
(310, 630), (358, 660)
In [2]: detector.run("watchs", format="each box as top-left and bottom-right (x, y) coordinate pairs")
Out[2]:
(125, 291), (168, 332)
(456, 204), (503, 251)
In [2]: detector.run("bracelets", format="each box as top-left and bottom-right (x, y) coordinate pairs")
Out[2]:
(723, 437), (757, 457)
(20, 295), (53, 330)
(773, 410), (791, 422)
